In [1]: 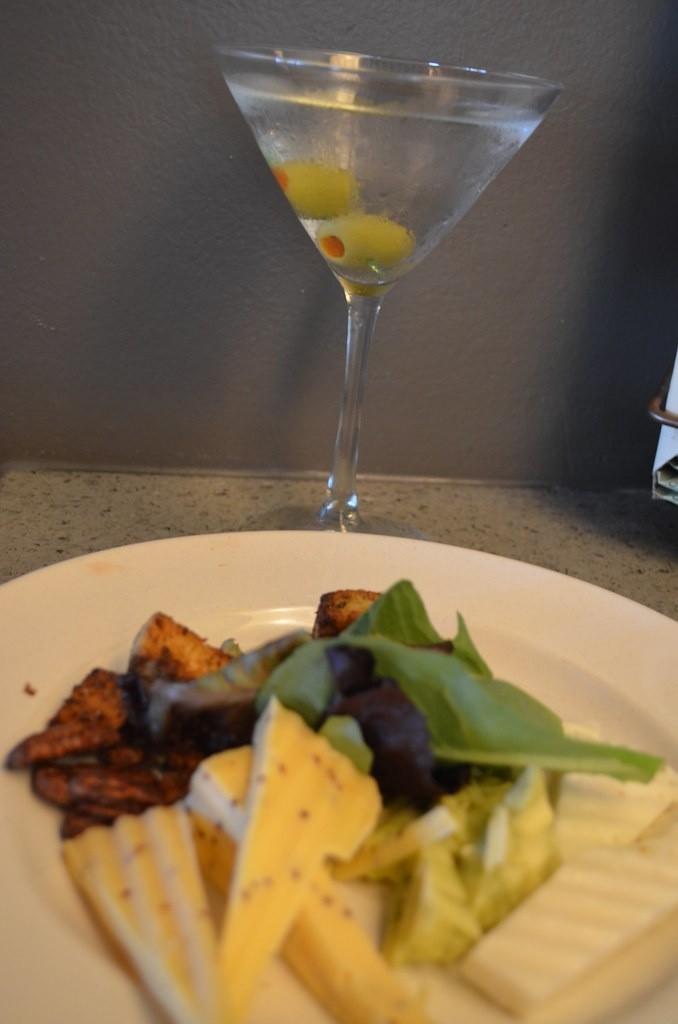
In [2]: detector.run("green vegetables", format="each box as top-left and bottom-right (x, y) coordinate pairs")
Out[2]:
(117, 577), (666, 809)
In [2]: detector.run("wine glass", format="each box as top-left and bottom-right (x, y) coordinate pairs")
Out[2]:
(212, 42), (562, 540)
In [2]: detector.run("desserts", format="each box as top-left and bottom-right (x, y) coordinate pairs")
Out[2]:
(64, 692), (446, 1022)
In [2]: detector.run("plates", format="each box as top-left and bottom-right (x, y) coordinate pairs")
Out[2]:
(2, 530), (678, 1023)
